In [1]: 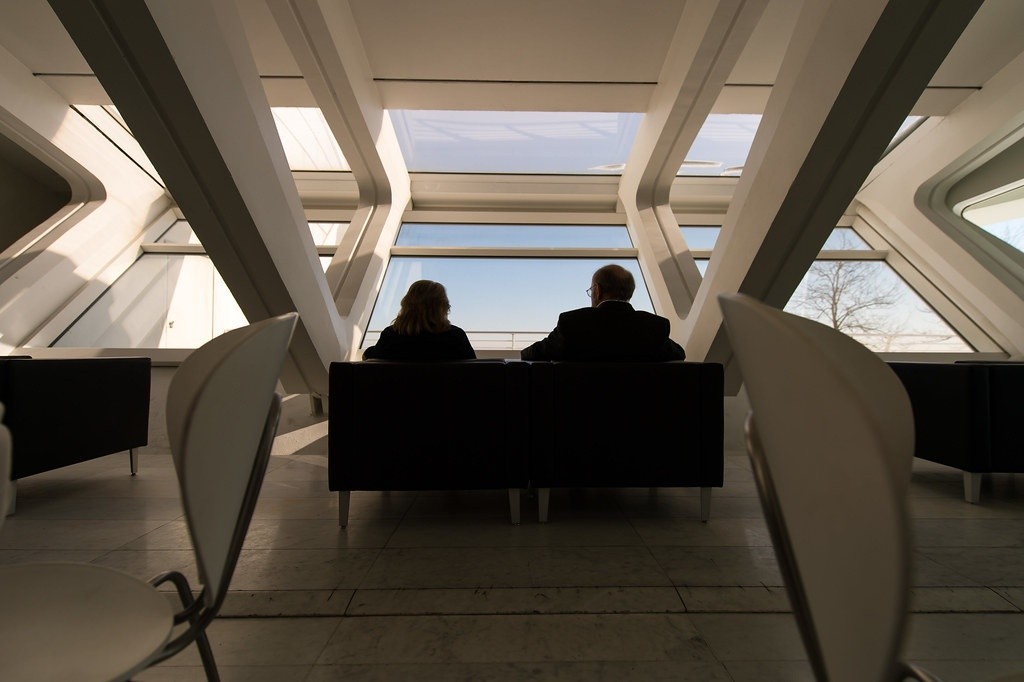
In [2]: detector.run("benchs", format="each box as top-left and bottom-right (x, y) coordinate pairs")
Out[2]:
(886, 361), (1024, 504)
(0, 356), (153, 517)
(328, 358), (726, 525)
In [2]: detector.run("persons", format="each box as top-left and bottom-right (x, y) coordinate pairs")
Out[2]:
(520, 264), (688, 361)
(361, 279), (479, 360)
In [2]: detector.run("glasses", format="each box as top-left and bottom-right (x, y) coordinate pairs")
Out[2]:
(586, 283), (600, 297)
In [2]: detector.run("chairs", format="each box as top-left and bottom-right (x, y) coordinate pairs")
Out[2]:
(0, 312), (300, 682)
(716, 292), (941, 682)
